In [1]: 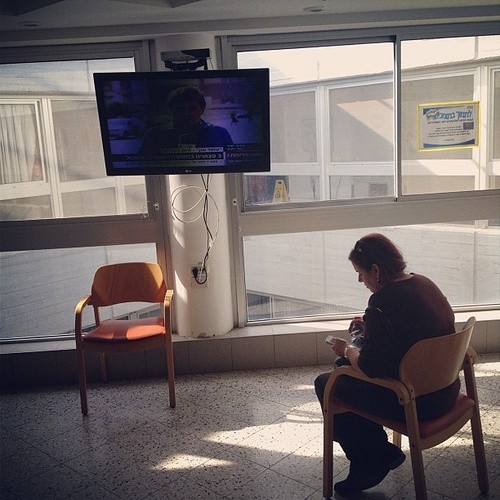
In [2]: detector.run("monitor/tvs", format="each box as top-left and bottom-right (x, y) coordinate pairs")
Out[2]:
(93, 68), (270, 176)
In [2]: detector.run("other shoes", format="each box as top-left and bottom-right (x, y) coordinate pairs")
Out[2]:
(334, 442), (406, 496)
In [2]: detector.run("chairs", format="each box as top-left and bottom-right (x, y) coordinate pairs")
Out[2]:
(74, 262), (178, 414)
(322, 316), (490, 498)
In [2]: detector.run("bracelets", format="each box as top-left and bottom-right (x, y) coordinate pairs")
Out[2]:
(344, 345), (359, 360)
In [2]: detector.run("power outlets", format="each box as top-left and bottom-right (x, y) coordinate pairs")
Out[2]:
(188, 260), (210, 290)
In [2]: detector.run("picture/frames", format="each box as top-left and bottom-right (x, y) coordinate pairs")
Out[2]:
(416, 99), (481, 152)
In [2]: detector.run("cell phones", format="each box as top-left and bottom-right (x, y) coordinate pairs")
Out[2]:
(326, 336), (334, 345)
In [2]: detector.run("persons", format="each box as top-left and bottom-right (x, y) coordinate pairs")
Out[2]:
(314, 233), (461, 493)
(137, 86), (234, 173)
